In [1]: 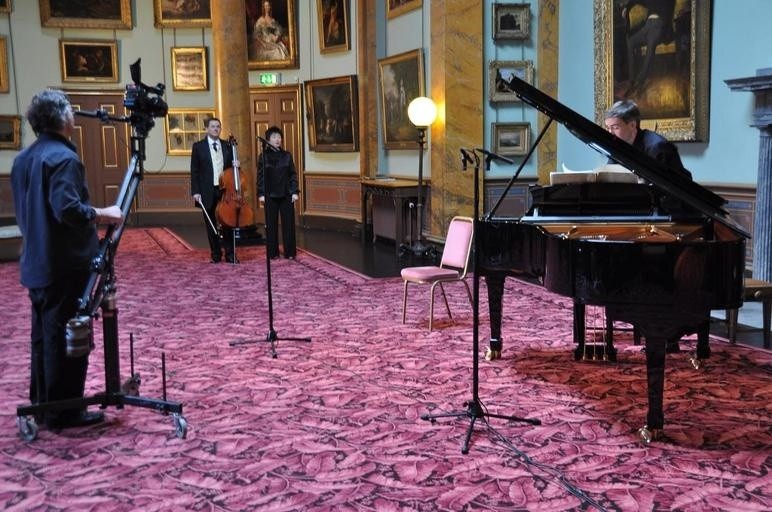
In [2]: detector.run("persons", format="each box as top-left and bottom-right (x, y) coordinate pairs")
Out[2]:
(619, 0), (674, 99)
(9, 86), (126, 431)
(596, 97), (692, 181)
(255, 125), (302, 260)
(250, 1), (289, 60)
(398, 78), (407, 122)
(69, 50), (106, 76)
(324, 4), (345, 44)
(88, 46), (110, 74)
(189, 117), (242, 264)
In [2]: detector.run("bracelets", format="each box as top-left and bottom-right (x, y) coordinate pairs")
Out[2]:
(99, 207), (104, 224)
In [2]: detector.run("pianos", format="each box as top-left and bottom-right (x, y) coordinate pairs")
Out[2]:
(473, 69), (751, 444)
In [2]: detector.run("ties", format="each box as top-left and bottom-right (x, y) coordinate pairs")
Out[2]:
(213, 143), (217, 150)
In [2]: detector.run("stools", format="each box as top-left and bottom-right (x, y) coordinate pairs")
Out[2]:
(0, 223), (23, 260)
(729, 279), (772, 349)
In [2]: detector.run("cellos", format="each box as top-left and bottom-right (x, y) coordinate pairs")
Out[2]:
(215, 135), (253, 229)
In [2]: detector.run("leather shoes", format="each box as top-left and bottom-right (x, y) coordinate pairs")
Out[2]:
(68, 411), (104, 428)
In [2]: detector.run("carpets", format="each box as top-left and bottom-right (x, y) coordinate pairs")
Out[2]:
(0, 224), (772, 512)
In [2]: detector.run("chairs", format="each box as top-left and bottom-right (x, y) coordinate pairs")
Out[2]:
(400, 213), (474, 332)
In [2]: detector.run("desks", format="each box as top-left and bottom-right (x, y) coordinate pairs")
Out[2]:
(359, 177), (429, 259)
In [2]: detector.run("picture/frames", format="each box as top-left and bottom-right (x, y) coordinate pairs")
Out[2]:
(590, 0), (714, 147)
(303, 73), (361, 154)
(161, 106), (218, 158)
(39, 0), (301, 96)
(384, 0), (424, 19)
(315, 0), (351, 55)
(0, 0), (20, 150)
(377, 48), (429, 151)
(490, 120), (532, 158)
(487, 58), (535, 104)
(489, 1), (532, 42)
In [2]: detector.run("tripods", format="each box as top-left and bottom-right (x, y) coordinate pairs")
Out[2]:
(421, 167), (541, 454)
(229, 143), (311, 358)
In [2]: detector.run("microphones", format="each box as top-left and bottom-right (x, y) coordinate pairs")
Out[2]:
(255, 135), (280, 153)
(458, 146), (480, 171)
(472, 147), (513, 171)
